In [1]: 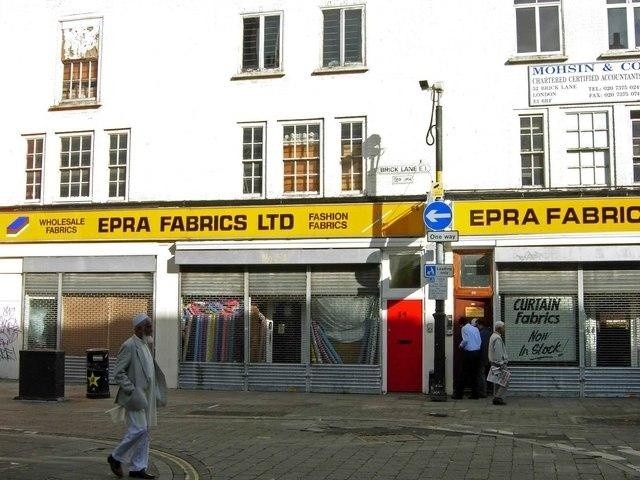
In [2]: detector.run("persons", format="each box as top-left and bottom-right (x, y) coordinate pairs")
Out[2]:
(107, 313), (169, 479)
(488, 321), (510, 407)
(475, 321), (492, 398)
(452, 317), (483, 400)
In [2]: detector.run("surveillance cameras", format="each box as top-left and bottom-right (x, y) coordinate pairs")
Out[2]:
(419, 80), (434, 91)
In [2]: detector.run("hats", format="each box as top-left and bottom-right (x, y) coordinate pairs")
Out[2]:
(130, 312), (149, 328)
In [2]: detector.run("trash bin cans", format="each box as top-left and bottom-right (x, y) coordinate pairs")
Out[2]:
(85, 348), (111, 399)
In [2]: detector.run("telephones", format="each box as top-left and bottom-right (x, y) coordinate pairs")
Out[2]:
(267, 320), (273, 331)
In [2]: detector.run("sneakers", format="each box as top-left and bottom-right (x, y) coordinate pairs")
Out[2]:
(449, 394), (508, 406)
(129, 468), (155, 480)
(106, 455), (124, 478)
